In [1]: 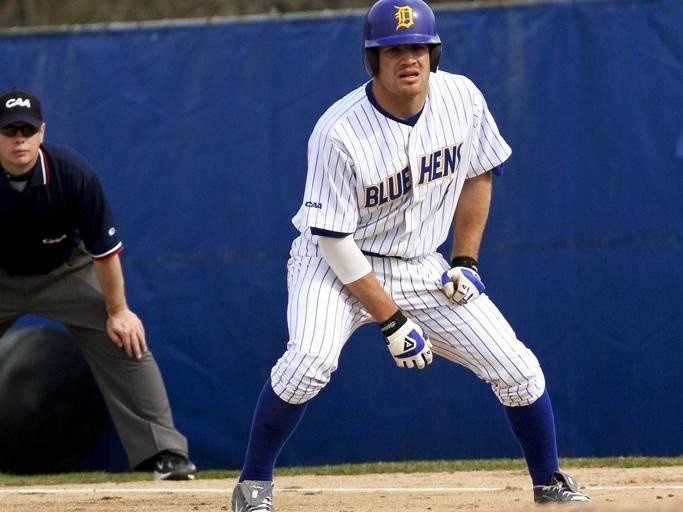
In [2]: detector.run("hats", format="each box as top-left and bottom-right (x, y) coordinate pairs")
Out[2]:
(0, 87), (42, 129)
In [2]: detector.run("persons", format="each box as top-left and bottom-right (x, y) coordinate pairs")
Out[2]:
(0, 88), (196, 481)
(232, 2), (589, 512)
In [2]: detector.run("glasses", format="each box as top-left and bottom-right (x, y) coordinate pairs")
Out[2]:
(0, 124), (39, 137)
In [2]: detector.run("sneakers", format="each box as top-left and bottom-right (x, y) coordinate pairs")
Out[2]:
(532, 473), (591, 504)
(231, 475), (273, 511)
(151, 449), (197, 480)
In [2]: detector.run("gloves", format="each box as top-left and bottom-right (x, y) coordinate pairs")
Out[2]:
(377, 307), (436, 369)
(440, 253), (486, 306)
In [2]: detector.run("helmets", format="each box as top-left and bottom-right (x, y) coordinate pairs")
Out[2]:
(362, 0), (441, 50)
